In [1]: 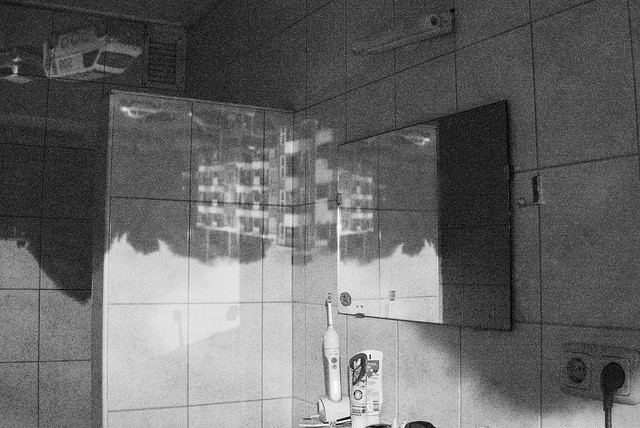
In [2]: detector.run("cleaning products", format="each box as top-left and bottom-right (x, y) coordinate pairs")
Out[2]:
(323, 294), (344, 402)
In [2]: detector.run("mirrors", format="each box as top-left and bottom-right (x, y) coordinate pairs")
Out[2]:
(337, 99), (514, 332)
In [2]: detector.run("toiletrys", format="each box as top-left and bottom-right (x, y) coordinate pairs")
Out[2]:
(362, 350), (384, 425)
(348, 354), (368, 428)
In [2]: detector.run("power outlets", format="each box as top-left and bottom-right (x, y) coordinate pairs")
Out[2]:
(559, 344), (636, 406)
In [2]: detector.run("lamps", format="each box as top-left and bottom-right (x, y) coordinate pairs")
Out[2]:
(352, 9), (454, 55)
